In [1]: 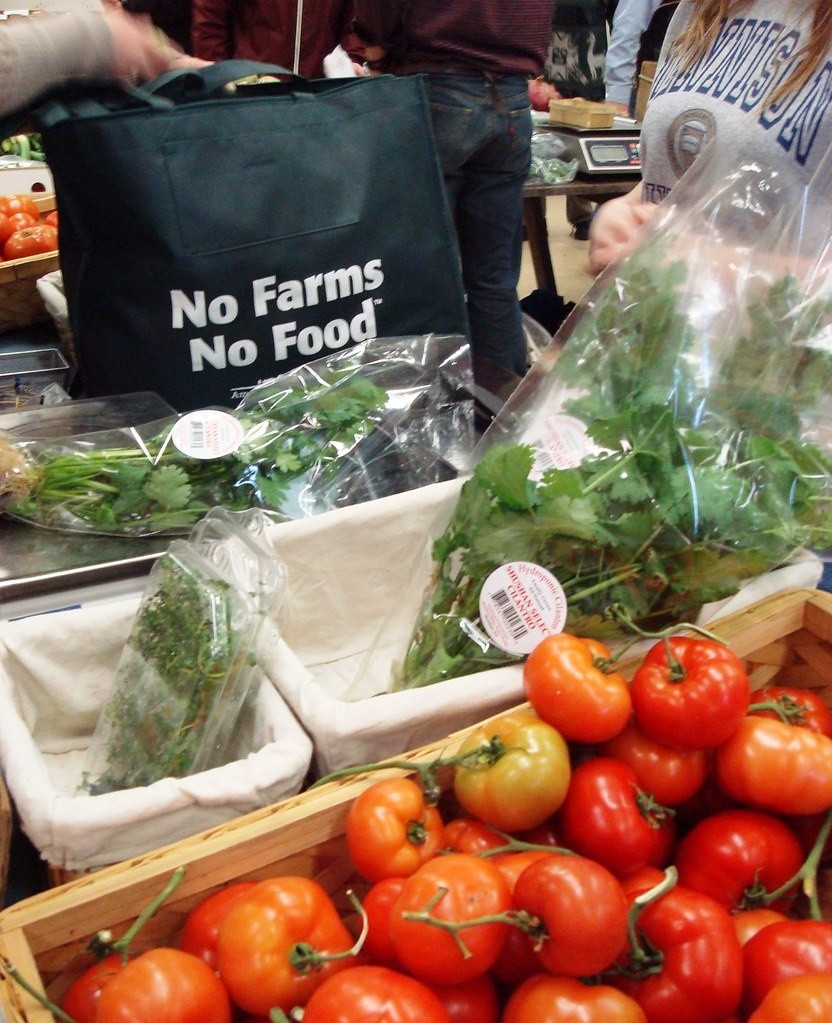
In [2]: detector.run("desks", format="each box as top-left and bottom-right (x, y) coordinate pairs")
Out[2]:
(523, 174), (642, 289)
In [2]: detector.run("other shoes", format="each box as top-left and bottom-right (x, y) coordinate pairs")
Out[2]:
(572, 219), (591, 240)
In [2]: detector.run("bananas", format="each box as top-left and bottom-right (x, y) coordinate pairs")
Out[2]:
(3, 133), (45, 161)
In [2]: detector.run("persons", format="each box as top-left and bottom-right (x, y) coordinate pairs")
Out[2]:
(0, 11), (257, 122)
(118, 0), (830, 432)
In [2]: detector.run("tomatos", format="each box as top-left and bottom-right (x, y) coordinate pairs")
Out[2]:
(0, 194), (59, 262)
(51, 631), (832, 1022)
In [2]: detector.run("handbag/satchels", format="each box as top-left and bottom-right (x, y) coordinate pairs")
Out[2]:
(44, 58), (475, 432)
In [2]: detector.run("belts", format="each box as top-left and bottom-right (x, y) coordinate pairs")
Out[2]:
(386, 62), (515, 77)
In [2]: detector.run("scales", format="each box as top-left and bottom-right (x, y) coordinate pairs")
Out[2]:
(540, 122), (651, 178)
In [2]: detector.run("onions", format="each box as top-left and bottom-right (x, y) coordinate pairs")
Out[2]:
(527, 75), (558, 111)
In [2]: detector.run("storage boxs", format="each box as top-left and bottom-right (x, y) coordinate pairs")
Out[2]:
(0, 589), (832, 1023)
(228, 475), (824, 779)
(0, 597), (315, 889)
(0, 194), (58, 336)
(547, 99), (620, 129)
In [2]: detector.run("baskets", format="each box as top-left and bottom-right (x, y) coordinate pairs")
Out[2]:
(0, 587), (832, 1022)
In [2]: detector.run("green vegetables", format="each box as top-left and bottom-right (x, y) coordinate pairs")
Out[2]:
(0, 260), (832, 804)
